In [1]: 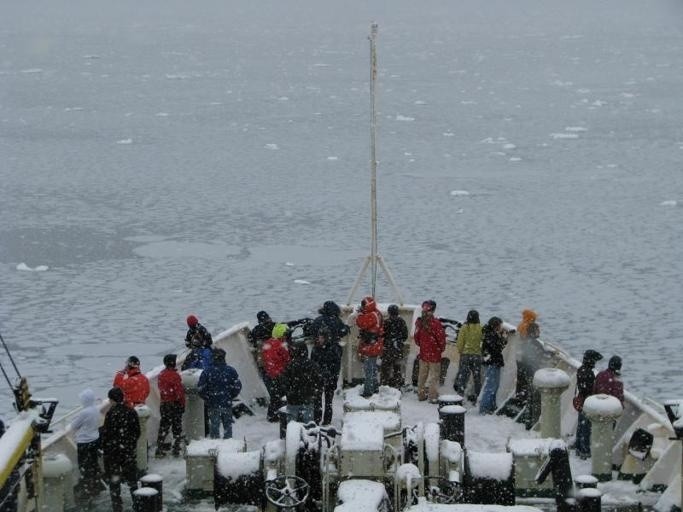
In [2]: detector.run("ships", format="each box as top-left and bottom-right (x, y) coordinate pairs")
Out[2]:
(0, 302), (682, 512)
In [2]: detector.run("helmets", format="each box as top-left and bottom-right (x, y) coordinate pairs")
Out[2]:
(164, 354), (177, 368)
(187, 315), (198, 326)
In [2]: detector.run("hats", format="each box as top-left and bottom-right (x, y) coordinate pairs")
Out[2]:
(109, 388), (123, 402)
(422, 300), (436, 313)
(316, 328), (333, 346)
(527, 323), (539, 335)
(293, 341), (308, 356)
(388, 305), (399, 316)
(125, 356), (140, 365)
(271, 323), (289, 339)
(608, 355), (622, 370)
(489, 317), (503, 326)
(467, 310), (479, 321)
(257, 311), (269, 323)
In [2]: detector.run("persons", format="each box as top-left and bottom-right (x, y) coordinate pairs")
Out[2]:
(260, 322), (292, 422)
(413, 300), (447, 404)
(380, 304), (407, 394)
(591, 354), (626, 430)
(99, 387), (143, 512)
(453, 309), (485, 405)
(303, 299), (352, 348)
(309, 326), (343, 427)
(183, 314), (212, 353)
(155, 353), (185, 458)
(244, 310), (291, 410)
(197, 348), (241, 438)
(70, 388), (100, 479)
(273, 339), (323, 428)
(514, 307), (538, 398)
(180, 332), (214, 437)
(478, 316), (508, 415)
(355, 295), (385, 399)
(572, 348), (602, 458)
(524, 323), (557, 431)
(112, 355), (149, 409)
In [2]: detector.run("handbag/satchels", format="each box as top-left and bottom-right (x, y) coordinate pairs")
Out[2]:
(358, 329), (377, 344)
(573, 397), (585, 413)
